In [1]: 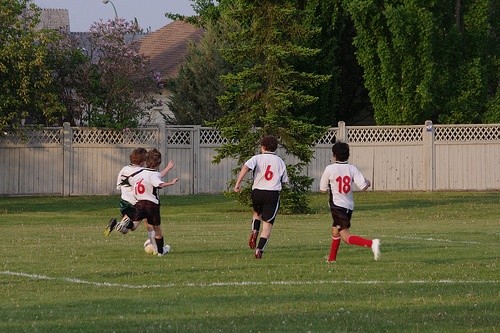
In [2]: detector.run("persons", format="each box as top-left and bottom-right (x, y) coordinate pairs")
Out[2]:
(234, 136), (288, 259)
(320, 141), (380, 263)
(102, 147), (179, 258)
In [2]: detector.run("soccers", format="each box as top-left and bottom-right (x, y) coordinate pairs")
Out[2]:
(144, 239), (153, 253)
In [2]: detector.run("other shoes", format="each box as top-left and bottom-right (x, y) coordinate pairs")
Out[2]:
(153, 249), (158, 256)
(158, 245), (171, 256)
(116, 217), (130, 232)
(104, 218), (116, 238)
(255, 248), (262, 259)
(370, 239), (380, 261)
(249, 230), (258, 249)
